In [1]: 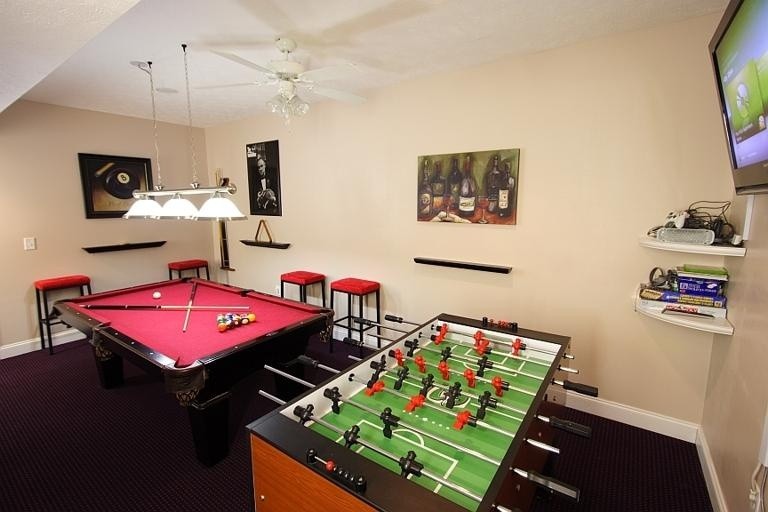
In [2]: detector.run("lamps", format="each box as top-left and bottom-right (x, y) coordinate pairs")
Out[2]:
(262, 78), (312, 122)
(119, 38), (250, 227)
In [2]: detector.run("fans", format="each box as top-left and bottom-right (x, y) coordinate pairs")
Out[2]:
(190, 30), (372, 106)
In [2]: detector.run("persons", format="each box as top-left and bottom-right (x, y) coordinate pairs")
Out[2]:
(255, 156), (278, 211)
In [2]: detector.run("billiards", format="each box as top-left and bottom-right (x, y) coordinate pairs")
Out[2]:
(103, 168), (140, 197)
(217, 312), (256, 331)
(153, 292), (162, 298)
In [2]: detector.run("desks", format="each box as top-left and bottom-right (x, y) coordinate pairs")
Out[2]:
(49, 273), (337, 471)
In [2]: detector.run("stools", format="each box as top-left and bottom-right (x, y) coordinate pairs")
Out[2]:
(280, 271), (326, 310)
(325, 277), (381, 355)
(33, 274), (93, 357)
(168, 259), (211, 280)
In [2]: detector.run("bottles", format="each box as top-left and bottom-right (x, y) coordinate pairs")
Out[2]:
(431, 161), (447, 214)
(486, 154), (516, 219)
(449, 152), (476, 216)
(419, 159), (435, 217)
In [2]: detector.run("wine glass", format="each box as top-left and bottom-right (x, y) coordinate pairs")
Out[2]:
(474, 194), (490, 223)
(439, 193), (455, 221)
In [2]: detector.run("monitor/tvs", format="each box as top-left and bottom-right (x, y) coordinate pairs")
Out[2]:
(707, 0), (768, 195)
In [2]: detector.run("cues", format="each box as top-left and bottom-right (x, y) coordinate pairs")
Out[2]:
(183, 282), (197, 331)
(216, 168), (229, 268)
(77, 305), (250, 309)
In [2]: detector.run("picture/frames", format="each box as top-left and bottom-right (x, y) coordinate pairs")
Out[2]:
(77, 152), (155, 219)
(246, 140), (283, 217)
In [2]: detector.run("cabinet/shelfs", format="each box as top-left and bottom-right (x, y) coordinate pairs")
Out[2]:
(626, 225), (747, 341)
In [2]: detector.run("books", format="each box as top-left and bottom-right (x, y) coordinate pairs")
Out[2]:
(635, 264), (730, 319)
